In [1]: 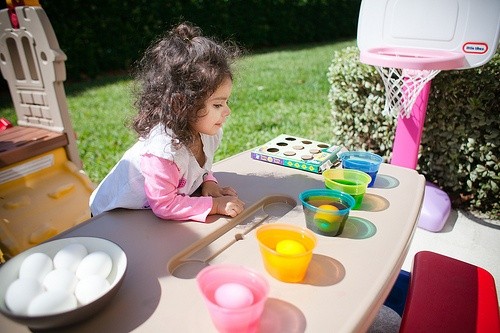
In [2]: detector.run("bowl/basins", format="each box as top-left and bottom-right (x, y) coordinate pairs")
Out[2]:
(255, 224), (319, 283)
(0, 236), (128, 329)
(340, 151), (383, 186)
(299, 189), (358, 236)
(322, 167), (373, 209)
(195, 263), (271, 333)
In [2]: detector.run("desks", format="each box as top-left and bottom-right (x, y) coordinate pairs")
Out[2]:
(0, 142), (426, 333)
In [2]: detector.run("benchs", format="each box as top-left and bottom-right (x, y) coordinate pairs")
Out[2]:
(382, 251), (500, 333)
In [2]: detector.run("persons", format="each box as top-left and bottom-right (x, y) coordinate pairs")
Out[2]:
(88, 25), (247, 222)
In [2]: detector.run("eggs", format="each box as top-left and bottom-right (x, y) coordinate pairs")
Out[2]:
(313, 204), (341, 231)
(276, 239), (305, 254)
(6, 243), (113, 316)
(214, 283), (254, 309)
